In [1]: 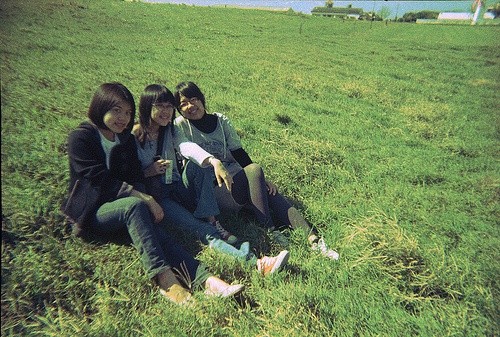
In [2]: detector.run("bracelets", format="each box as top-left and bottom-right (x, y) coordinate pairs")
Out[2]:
(208, 156), (214, 164)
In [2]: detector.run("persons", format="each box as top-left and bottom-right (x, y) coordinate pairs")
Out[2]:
(134, 84), (289, 276)
(60, 81), (245, 307)
(131, 81), (340, 261)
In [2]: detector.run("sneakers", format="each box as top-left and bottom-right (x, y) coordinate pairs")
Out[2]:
(312, 238), (340, 259)
(209, 220), (237, 244)
(158, 284), (194, 306)
(205, 283), (245, 299)
(267, 228), (289, 246)
(257, 250), (290, 276)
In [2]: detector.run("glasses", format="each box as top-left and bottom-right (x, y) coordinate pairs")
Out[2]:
(177, 97), (200, 108)
(152, 104), (175, 111)
